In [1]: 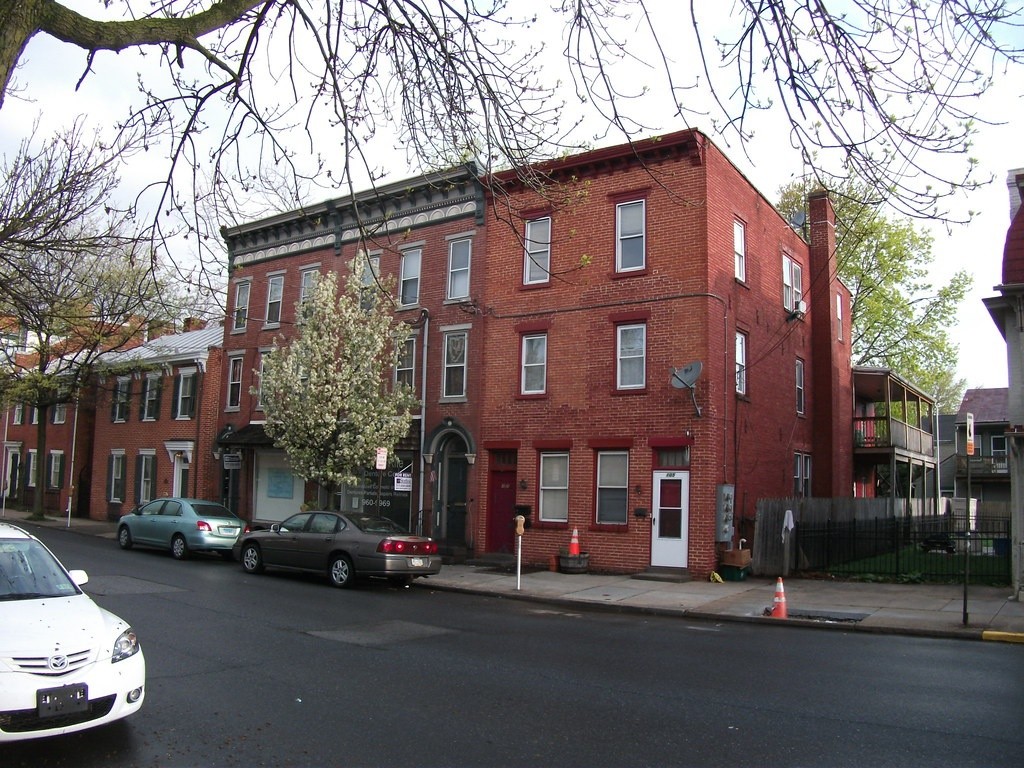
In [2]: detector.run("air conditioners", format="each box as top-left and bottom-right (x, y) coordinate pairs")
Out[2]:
(795, 300), (806, 314)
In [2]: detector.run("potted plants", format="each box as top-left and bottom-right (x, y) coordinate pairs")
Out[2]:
(558, 547), (590, 574)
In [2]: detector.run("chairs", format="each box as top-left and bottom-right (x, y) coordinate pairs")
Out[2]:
(981, 456), (997, 473)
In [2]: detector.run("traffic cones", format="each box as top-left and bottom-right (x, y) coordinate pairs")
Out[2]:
(568, 526), (580, 555)
(770, 577), (788, 618)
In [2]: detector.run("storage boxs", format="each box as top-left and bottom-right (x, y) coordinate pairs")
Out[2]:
(719, 564), (749, 582)
(724, 549), (752, 564)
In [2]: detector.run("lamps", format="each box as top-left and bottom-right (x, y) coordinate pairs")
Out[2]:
(518, 479), (528, 490)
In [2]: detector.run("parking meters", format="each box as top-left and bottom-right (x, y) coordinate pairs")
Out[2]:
(67, 485), (75, 527)
(2, 481), (8, 517)
(514, 516), (525, 590)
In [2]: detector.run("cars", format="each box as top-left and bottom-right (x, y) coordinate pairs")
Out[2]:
(116, 497), (251, 560)
(233, 510), (442, 588)
(0, 523), (146, 743)
